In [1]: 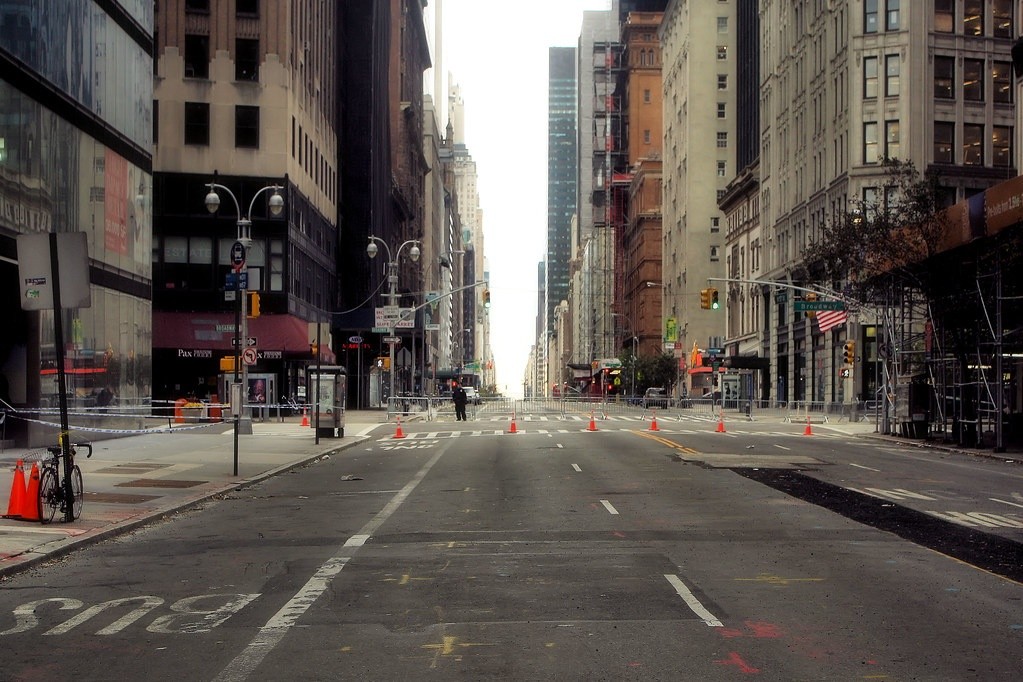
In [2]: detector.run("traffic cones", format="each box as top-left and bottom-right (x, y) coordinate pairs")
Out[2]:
(714, 411), (727, 433)
(17, 462), (49, 521)
(390, 416), (408, 438)
(0, 458), (27, 519)
(299, 406), (311, 426)
(507, 413), (520, 433)
(586, 408), (600, 432)
(801, 414), (816, 436)
(648, 410), (661, 431)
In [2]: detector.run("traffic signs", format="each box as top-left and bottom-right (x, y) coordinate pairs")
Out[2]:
(381, 335), (404, 345)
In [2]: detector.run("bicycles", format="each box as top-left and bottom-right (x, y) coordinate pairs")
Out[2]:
(35, 443), (93, 525)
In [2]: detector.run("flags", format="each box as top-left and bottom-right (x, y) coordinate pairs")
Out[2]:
(815, 310), (846, 332)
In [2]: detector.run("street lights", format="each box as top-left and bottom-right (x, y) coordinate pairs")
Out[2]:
(367, 236), (422, 416)
(448, 328), (473, 399)
(647, 282), (681, 400)
(611, 312), (635, 401)
(202, 180), (287, 433)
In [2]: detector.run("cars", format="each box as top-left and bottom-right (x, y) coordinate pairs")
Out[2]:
(462, 386), (482, 405)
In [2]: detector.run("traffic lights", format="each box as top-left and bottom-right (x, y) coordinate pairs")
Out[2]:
(484, 288), (492, 308)
(605, 368), (609, 376)
(804, 293), (817, 319)
(843, 342), (854, 364)
(252, 293), (262, 319)
(609, 386), (612, 391)
(452, 381), (457, 390)
(839, 367), (850, 378)
(700, 289), (711, 311)
(709, 289), (719, 311)
(310, 346), (317, 356)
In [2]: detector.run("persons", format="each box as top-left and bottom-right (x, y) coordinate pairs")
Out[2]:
(452, 384), (467, 421)
(97, 384), (113, 414)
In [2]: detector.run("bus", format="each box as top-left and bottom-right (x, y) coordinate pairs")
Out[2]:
(551, 383), (568, 401)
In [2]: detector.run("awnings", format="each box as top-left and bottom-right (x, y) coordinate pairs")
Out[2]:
(290, 315), (336, 363)
(152, 314), (311, 359)
(723, 356), (770, 369)
(428, 371), (458, 379)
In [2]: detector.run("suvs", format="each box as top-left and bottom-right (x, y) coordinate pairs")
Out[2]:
(642, 387), (667, 409)
